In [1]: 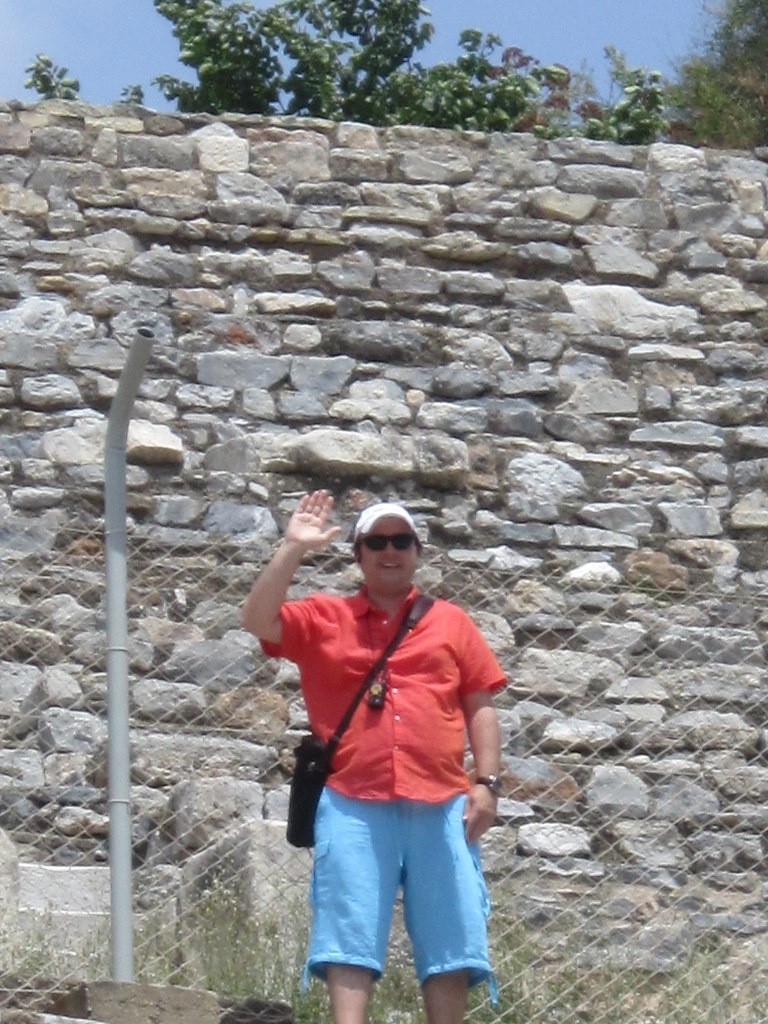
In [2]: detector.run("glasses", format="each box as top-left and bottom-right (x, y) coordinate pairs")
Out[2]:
(358, 533), (416, 551)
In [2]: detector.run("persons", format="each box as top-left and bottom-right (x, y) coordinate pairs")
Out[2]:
(240, 488), (509, 1024)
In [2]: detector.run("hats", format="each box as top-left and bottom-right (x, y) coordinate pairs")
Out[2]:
(354, 503), (417, 543)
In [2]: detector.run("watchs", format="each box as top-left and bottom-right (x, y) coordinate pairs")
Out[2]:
(476, 775), (504, 796)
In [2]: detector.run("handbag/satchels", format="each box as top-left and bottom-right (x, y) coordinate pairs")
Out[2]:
(287, 734), (331, 848)
